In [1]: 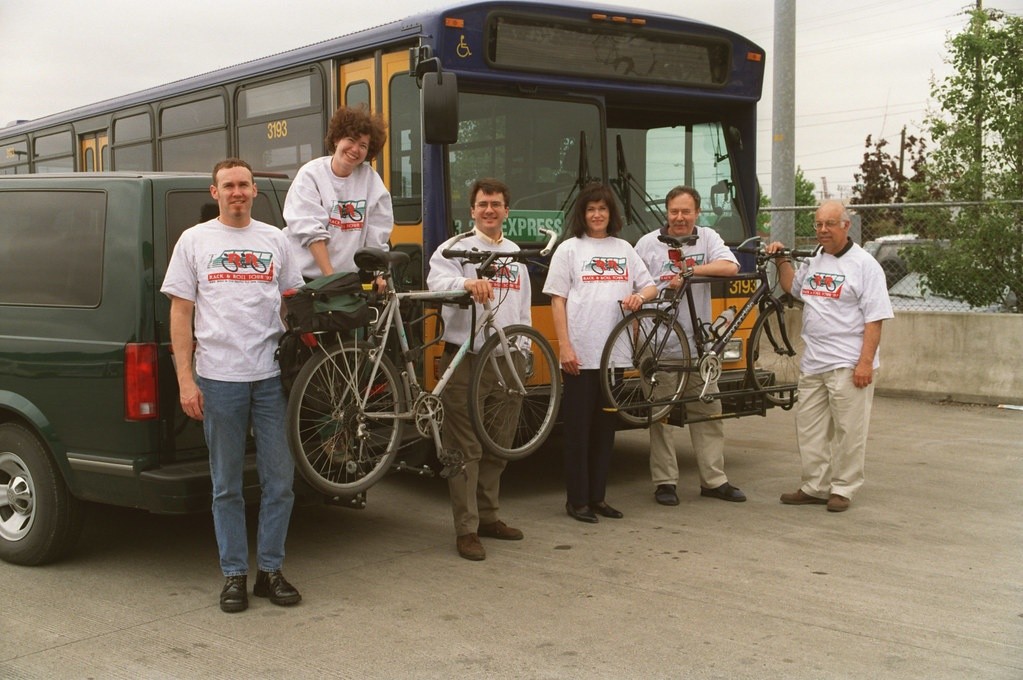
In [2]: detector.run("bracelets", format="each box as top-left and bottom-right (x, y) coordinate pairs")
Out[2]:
(636, 293), (647, 304)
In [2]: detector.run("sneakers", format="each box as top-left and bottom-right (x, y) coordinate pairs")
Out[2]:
(456, 533), (486, 560)
(701, 482), (746, 502)
(219, 575), (248, 612)
(654, 484), (680, 506)
(780, 489), (829, 505)
(254, 570), (303, 605)
(478, 520), (523, 540)
(827, 495), (849, 511)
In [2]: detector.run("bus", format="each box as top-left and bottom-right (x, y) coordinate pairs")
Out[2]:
(0, 0), (776, 418)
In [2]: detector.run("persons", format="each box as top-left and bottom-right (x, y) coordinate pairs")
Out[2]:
(159, 158), (308, 612)
(278, 106), (394, 466)
(426, 181), (524, 561)
(631, 186), (746, 505)
(766, 199), (894, 511)
(542, 186), (659, 523)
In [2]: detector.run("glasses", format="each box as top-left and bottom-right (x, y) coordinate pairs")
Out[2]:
(813, 221), (846, 230)
(471, 201), (511, 209)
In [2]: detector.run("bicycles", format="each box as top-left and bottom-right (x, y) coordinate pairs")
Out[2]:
(277, 227), (563, 500)
(598, 234), (818, 429)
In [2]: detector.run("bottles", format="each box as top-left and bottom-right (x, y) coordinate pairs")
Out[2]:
(711, 306), (737, 336)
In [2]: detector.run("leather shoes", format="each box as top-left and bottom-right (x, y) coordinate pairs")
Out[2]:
(566, 501), (598, 523)
(593, 500), (623, 518)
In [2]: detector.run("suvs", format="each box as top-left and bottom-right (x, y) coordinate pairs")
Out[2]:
(0, 168), (293, 568)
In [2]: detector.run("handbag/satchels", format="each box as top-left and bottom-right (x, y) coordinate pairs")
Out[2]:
(273, 330), (312, 377)
(282, 271), (371, 332)
